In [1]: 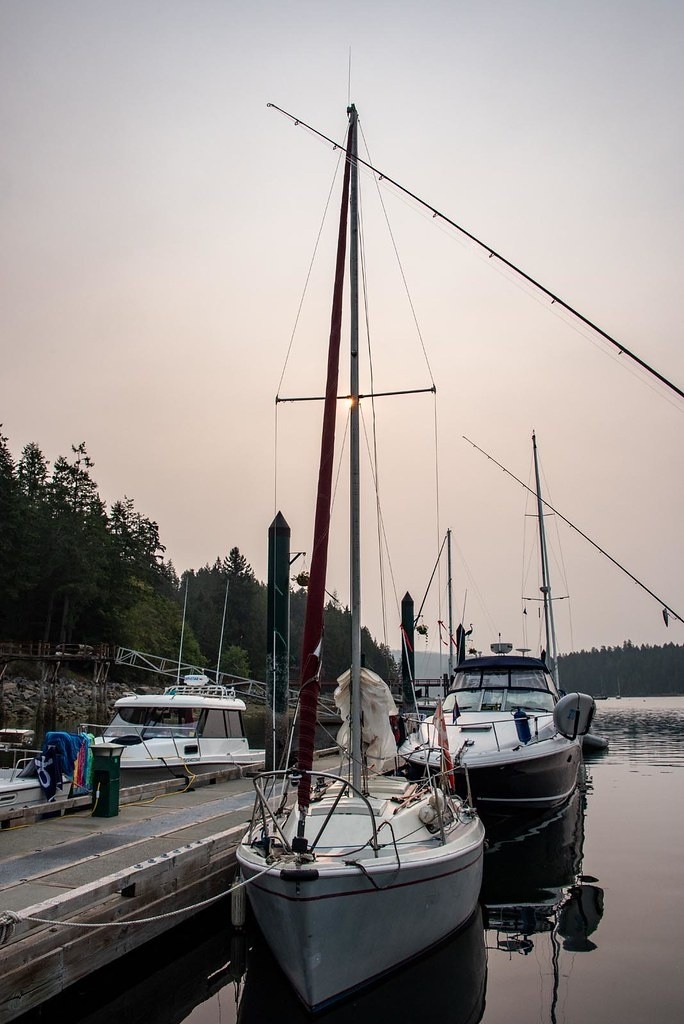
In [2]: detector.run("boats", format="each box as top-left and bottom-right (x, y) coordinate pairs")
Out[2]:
(388, 427), (594, 813)
(474, 788), (586, 961)
(0, 728), (78, 815)
(75, 578), (266, 792)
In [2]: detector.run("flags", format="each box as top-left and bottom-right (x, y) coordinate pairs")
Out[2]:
(453, 702), (461, 723)
(433, 701), (455, 792)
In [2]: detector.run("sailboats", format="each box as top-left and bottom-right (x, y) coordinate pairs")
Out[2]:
(235, 107), (486, 1015)
